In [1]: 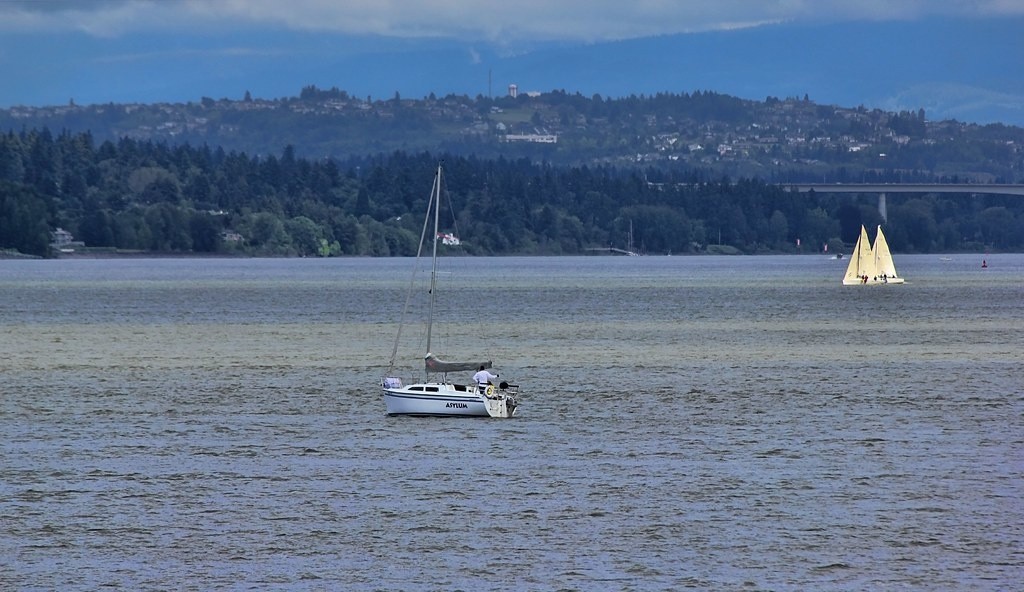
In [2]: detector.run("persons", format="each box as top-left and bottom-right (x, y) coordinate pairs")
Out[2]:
(860, 273), (896, 284)
(472, 366), (499, 394)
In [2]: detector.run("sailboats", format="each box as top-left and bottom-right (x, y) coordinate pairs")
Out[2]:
(841, 224), (905, 285)
(376, 156), (522, 419)
